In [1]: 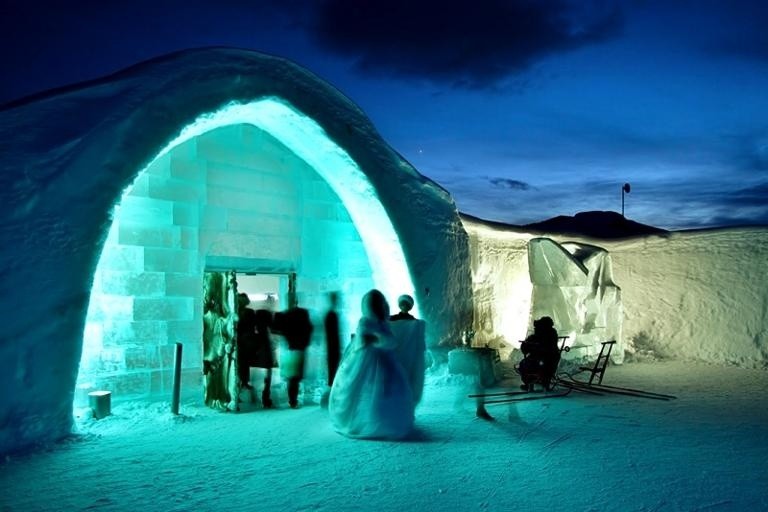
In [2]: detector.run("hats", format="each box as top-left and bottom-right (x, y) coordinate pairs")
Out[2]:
(397, 292), (415, 310)
(532, 317), (554, 330)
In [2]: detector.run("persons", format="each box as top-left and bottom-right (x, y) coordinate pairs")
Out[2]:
(389, 295), (415, 320)
(517, 316), (561, 392)
(249, 295), (279, 407)
(234, 293), (256, 390)
(277, 291), (314, 409)
(328, 288), (428, 441)
(323, 290), (341, 385)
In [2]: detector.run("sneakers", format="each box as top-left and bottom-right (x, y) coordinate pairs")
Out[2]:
(475, 408), (496, 423)
(237, 380), (304, 410)
(519, 383), (552, 394)
